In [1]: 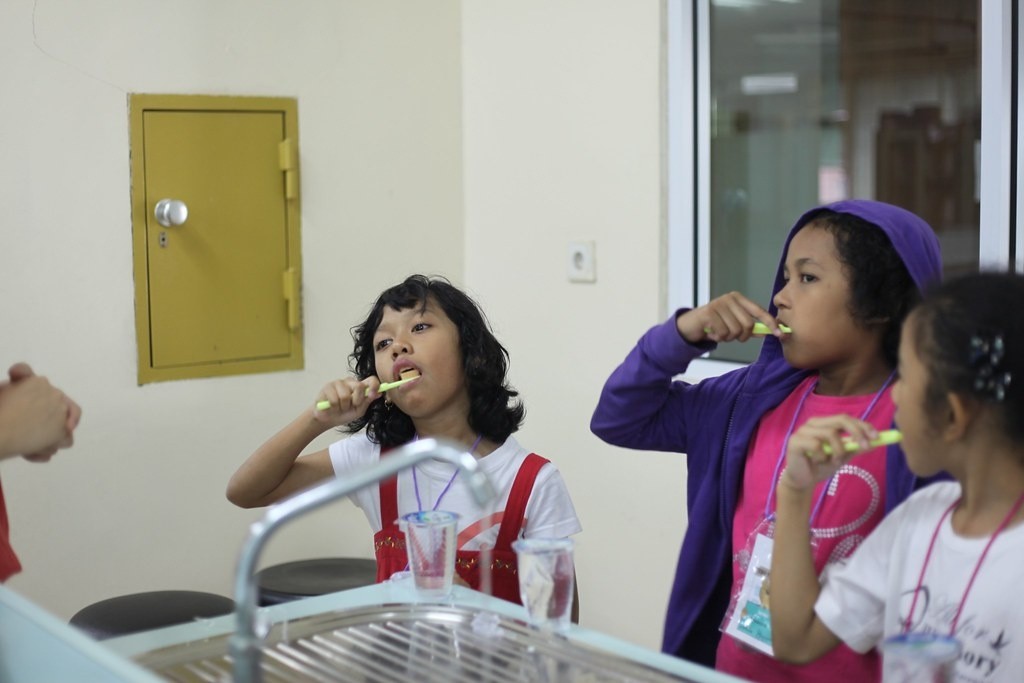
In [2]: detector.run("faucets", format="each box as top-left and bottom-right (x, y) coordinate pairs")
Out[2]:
(228, 437), (497, 683)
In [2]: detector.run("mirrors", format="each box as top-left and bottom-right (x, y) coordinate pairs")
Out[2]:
(665, 0), (1012, 388)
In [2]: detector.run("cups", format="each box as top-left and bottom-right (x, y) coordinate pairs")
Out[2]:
(513, 538), (576, 639)
(402, 508), (463, 599)
(877, 633), (963, 683)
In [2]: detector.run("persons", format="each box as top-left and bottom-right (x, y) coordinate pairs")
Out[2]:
(1, 363), (84, 585)
(766, 263), (1022, 683)
(226, 273), (582, 626)
(591, 198), (949, 683)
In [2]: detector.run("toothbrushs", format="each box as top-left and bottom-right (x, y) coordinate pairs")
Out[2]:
(316, 370), (422, 412)
(804, 429), (903, 458)
(702, 322), (792, 335)
